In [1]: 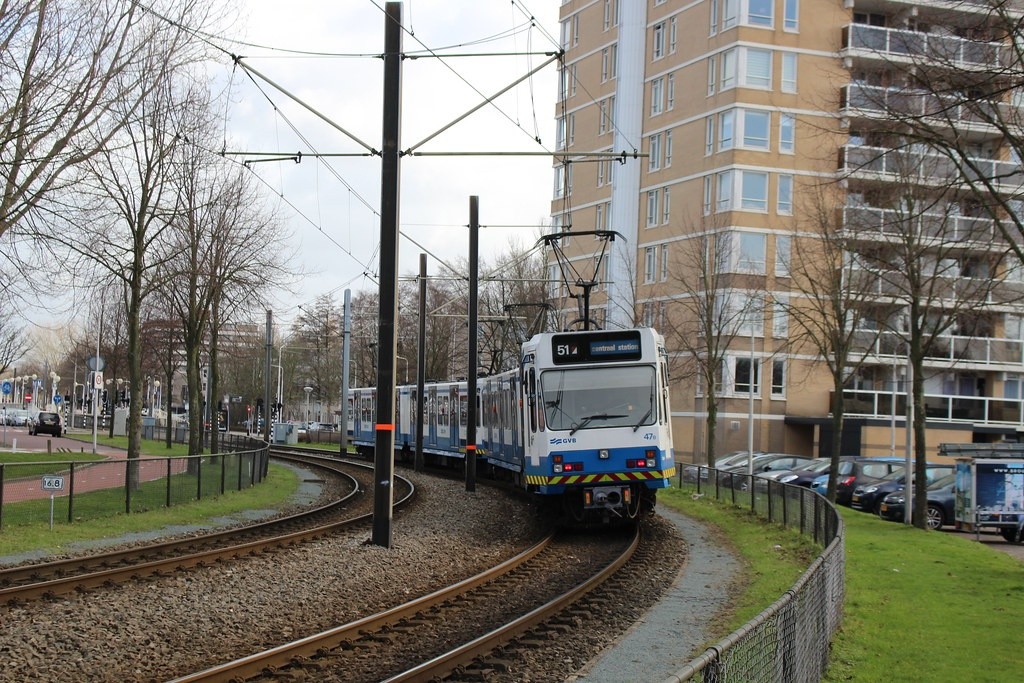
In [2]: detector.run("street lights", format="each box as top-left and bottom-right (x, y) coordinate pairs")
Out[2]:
(303, 387), (314, 431)
(396, 355), (409, 384)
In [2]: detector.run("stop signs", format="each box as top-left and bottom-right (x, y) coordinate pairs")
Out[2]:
(25, 394), (32, 402)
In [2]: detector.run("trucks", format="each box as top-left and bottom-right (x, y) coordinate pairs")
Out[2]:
(955, 456), (1024, 543)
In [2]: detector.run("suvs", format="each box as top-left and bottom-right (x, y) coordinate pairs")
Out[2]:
(28, 411), (62, 437)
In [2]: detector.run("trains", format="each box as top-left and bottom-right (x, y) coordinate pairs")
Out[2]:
(347, 229), (677, 525)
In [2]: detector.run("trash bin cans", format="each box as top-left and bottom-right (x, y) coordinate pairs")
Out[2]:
(286, 424), (299, 444)
(318, 423), (332, 444)
(309, 429), (318, 442)
(331, 431), (342, 443)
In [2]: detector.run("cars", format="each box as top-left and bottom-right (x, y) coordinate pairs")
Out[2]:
(0, 409), (31, 427)
(849, 461), (957, 512)
(878, 473), (958, 531)
(286, 420), (334, 433)
(684, 449), (935, 506)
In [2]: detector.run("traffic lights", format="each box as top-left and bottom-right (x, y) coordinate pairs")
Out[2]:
(102, 389), (108, 404)
(256, 398), (264, 412)
(120, 390), (126, 404)
(278, 403), (282, 410)
(122, 397), (130, 404)
